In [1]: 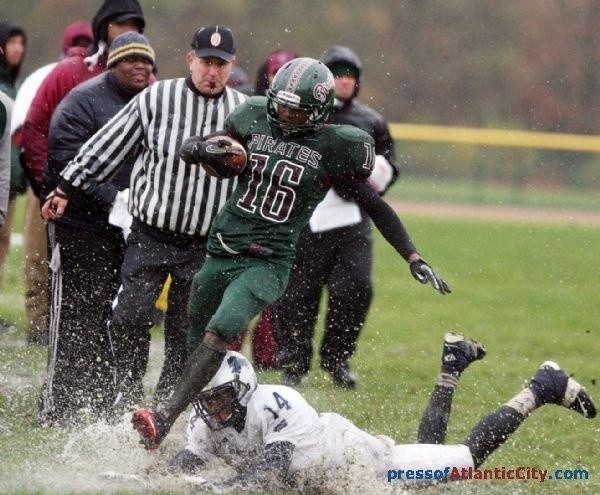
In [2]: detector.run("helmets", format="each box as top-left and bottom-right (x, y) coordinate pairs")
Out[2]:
(191, 350), (257, 432)
(266, 57), (335, 142)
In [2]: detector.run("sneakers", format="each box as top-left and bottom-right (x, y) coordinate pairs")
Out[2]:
(530, 360), (596, 419)
(321, 358), (356, 386)
(442, 331), (486, 373)
(130, 410), (170, 451)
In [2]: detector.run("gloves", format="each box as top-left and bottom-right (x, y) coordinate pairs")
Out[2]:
(410, 258), (451, 295)
(192, 139), (242, 179)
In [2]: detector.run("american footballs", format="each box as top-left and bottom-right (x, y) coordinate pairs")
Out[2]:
(201, 136), (246, 178)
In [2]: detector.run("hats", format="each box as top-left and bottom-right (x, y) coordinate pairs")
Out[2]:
(107, 31), (155, 70)
(92, 0), (145, 37)
(191, 25), (236, 63)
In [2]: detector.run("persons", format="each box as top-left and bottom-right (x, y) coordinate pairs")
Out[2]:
(1, 1), (452, 450)
(103, 333), (600, 494)
